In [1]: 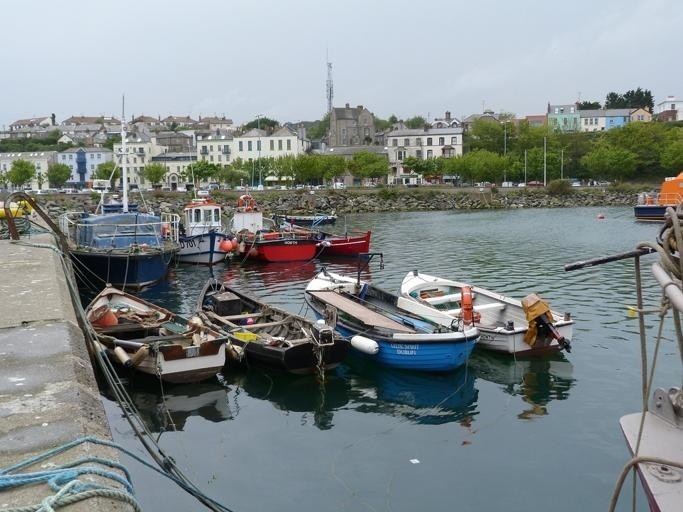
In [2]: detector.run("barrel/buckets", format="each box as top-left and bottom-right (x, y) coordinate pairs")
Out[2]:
(233, 332), (257, 340)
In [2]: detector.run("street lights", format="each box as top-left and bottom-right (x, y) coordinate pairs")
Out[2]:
(253, 112), (267, 190)
(499, 120), (510, 182)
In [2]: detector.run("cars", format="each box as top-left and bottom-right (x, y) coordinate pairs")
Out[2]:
(568, 176), (617, 187)
(364, 181), (433, 189)
(445, 178), (546, 188)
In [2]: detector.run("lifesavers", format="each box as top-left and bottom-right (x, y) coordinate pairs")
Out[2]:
(461, 286), (473, 325)
(238, 194), (254, 211)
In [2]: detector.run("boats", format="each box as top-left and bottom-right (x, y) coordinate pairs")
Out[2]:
(272, 213), (376, 259)
(221, 175), (316, 265)
(395, 259), (576, 365)
(264, 202), (343, 230)
(630, 170), (683, 224)
(83, 277), (231, 389)
(44, 89), (185, 298)
(302, 263), (483, 377)
(156, 185), (236, 271)
(191, 273), (355, 385)
